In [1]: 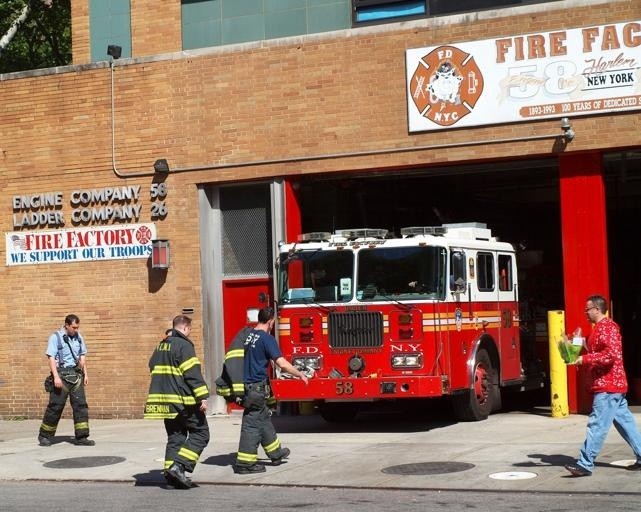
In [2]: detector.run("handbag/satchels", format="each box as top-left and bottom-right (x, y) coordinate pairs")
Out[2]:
(45, 376), (55, 392)
(242, 390), (266, 410)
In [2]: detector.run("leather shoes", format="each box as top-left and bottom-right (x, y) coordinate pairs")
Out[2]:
(565, 464), (592, 477)
(626, 463), (641, 471)
(38, 435), (52, 446)
(166, 461), (194, 490)
(234, 462), (267, 474)
(160, 474), (192, 488)
(73, 439), (95, 447)
(271, 448), (290, 466)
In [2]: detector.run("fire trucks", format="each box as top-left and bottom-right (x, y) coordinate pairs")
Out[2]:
(273, 223), (525, 419)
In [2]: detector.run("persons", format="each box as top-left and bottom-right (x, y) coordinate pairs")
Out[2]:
(143, 314), (210, 488)
(215, 306), (309, 475)
(565, 295), (641, 477)
(38, 314), (95, 447)
